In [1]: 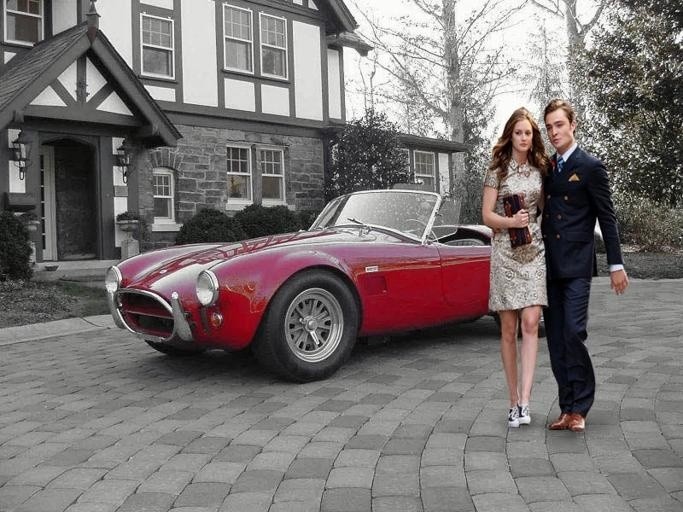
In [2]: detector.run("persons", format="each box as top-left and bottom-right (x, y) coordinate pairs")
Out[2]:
(537, 97), (630, 433)
(479, 104), (555, 432)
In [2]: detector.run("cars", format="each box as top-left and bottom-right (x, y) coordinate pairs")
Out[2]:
(104, 189), (548, 380)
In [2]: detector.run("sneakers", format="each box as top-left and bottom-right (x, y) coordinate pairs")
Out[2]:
(505, 402), (532, 428)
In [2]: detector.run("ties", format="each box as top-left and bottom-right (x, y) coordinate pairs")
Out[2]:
(556, 157), (564, 172)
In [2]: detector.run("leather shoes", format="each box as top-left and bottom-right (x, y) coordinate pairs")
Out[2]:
(548, 411), (587, 432)
